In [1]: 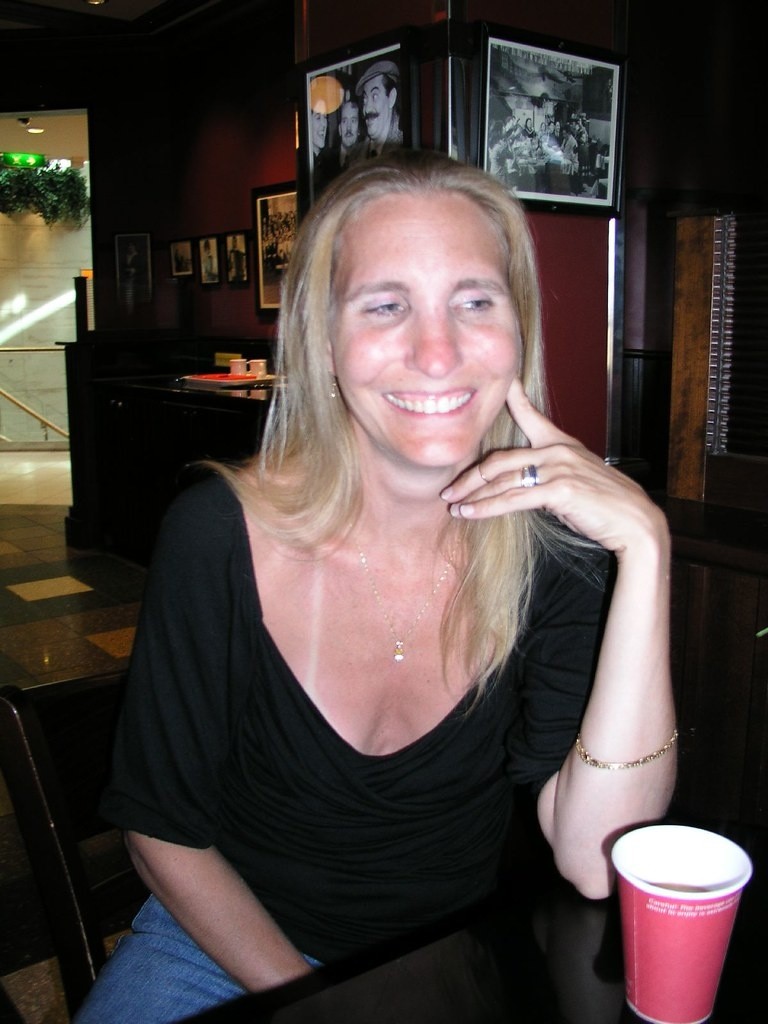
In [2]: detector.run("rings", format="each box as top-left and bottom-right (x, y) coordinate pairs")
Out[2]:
(521, 464), (538, 488)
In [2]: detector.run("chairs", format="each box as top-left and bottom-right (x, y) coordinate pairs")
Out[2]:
(0, 562), (715, 1024)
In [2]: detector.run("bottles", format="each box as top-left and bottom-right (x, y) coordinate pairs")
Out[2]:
(230, 359), (248, 397)
(249, 359), (268, 400)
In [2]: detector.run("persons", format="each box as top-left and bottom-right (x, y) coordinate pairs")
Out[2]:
(487, 112), (590, 191)
(120, 208), (299, 301)
(331, 100), (364, 172)
(71, 150), (679, 1023)
(345, 60), (412, 169)
(310, 99), (342, 200)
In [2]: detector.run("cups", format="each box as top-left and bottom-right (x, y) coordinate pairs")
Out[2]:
(610, 824), (752, 1024)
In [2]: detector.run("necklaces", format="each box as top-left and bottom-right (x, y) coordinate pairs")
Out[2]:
(350, 520), (461, 664)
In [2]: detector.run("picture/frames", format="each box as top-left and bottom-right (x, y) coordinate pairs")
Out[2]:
(298, 23), (419, 223)
(223, 230), (251, 286)
(197, 233), (224, 289)
(251, 180), (297, 319)
(168, 236), (199, 283)
(469, 20), (629, 220)
(114, 234), (153, 285)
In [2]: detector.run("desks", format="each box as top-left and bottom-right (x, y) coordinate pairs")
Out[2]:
(176, 819), (768, 1024)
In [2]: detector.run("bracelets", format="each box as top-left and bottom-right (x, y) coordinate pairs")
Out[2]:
(574, 728), (680, 771)
